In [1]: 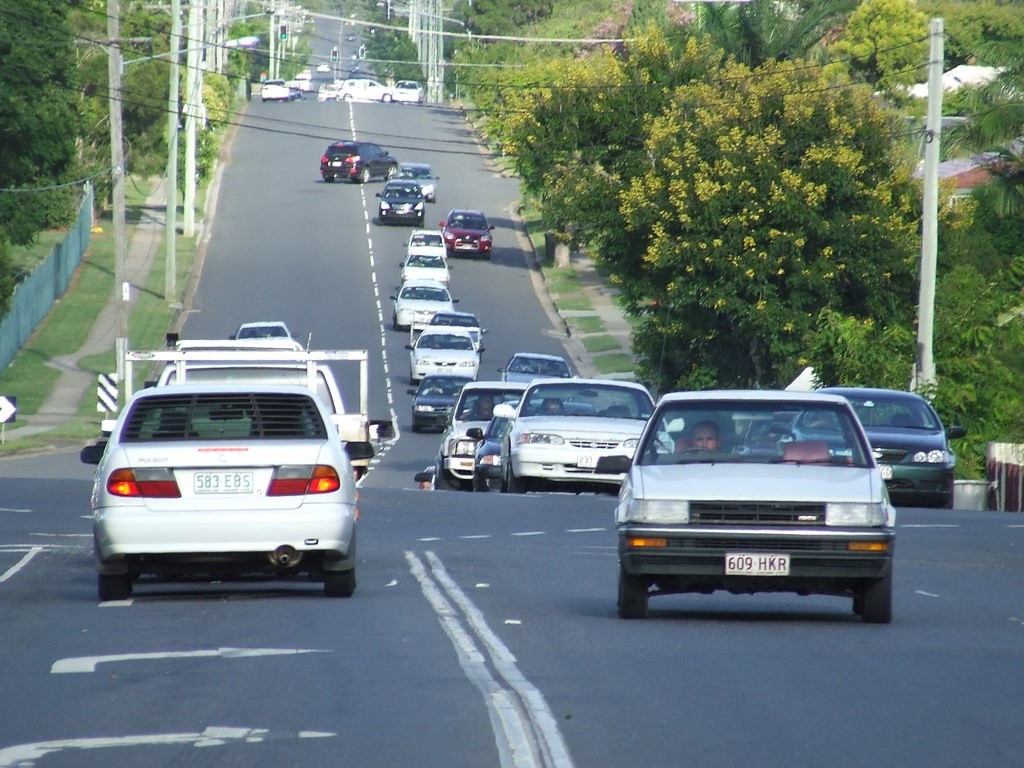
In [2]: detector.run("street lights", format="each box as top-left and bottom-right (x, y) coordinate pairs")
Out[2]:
(376, 3), (474, 43)
(107, 36), (261, 425)
(164, 6), (276, 302)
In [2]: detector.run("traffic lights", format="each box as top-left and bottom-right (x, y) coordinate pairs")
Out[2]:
(280, 24), (288, 39)
(331, 50), (339, 62)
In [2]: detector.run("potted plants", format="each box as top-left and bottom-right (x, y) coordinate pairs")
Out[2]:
(952, 455), (990, 510)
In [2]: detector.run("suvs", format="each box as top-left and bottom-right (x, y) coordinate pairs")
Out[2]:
(319, 139), (398, 183)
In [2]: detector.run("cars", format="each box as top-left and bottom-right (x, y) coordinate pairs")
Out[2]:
(388, 161), (440, 204)
(337, 79), (394, 103)
(466, 399), (598, 492)
(613, 388), (899, 624)
(375, 180), (429, 227)
(496, 352), (572, 406)
(262, 63), (337, 102)
(438, 208), (497, 260)
(492, 376), (658, 498)
(406, 375), (475, 435)
(815, 386), (965, 510)
(82, 321), (395, 604)
(392, 79), (427, 104)
(402, 229), (452, 260)
(389, 280), (460, 332)
(398, 253), (454, 290)
(405, 310), (486, 382)
(412, 381), (541, 494)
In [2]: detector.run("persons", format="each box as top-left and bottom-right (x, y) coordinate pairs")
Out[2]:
(691, 419), (735, 463)
(545, 362), (565, 377)
(385, 189), (400, 198)
(517, 357), (535, 372)
(409, 258), (426, 267)
(451, 219), (460, 229)
(404, 289), (419, 298)
(538, 397), (561, 416)
(441, 320), (451, 326)
(422, 338), (438, 348)
(413, 238), (426, 246)
(468, 392), (495, 422)
(400, 169), (413, 179)
(422, 380), (444, 396)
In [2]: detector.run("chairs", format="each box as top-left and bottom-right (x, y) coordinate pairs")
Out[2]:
(152, 412), (193, 438)
(250, 413), (307, 437)
(671, 436), (693, 454)
(605, 405), (629, 417)
(783, 441), (830, 464)
(893, 413), (912, 427)
